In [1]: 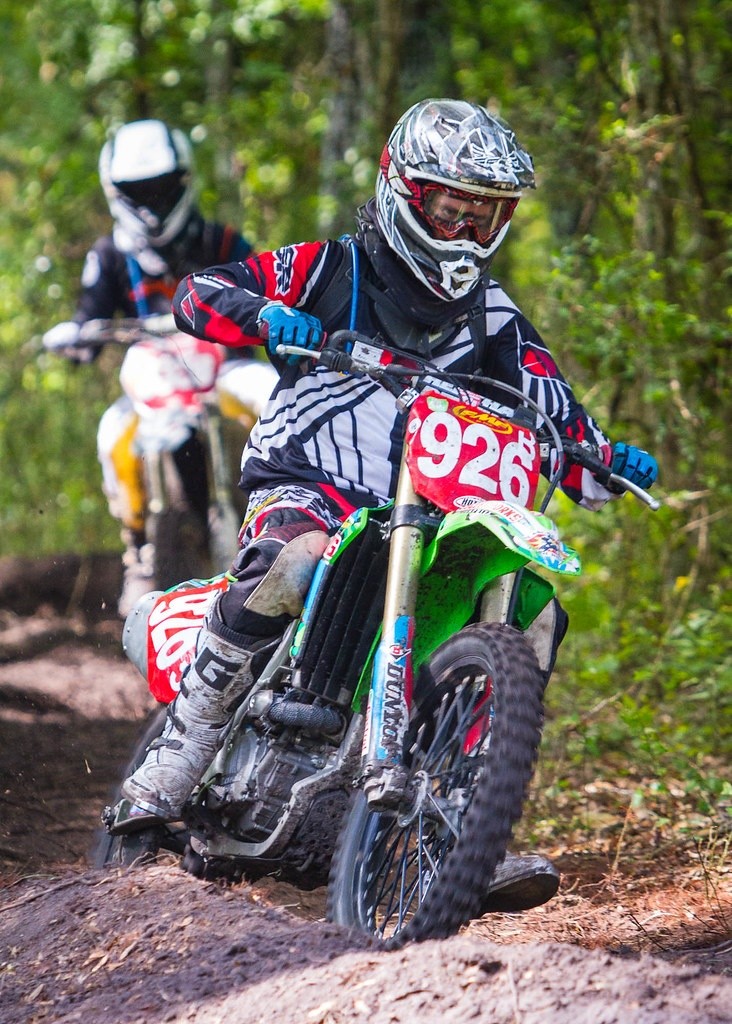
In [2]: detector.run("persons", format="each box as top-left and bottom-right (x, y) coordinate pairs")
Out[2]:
(61, 119), (260, 619)
(119, 96), (659, 921)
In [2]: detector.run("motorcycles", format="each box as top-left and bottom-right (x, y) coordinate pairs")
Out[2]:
(34, 312), (259, 592)
(95, 321), (659, 952)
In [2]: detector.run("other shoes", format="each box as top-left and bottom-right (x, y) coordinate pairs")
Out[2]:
(117, 564), (158, 619)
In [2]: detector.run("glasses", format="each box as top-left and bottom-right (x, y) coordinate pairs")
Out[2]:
(422, 183), (521, 244)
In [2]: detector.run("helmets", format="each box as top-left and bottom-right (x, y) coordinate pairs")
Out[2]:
(97, 119), (199, 247)
(374, 97), (538, 303)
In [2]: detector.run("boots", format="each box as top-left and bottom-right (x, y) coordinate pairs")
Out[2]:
(118, 591), (285, 822)
(419, 852), (560, 910)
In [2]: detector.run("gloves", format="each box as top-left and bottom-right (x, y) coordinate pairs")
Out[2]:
(256, 300), (323, 370)
(606, 440), (659, 494)
(42, 321), (81, 350)
